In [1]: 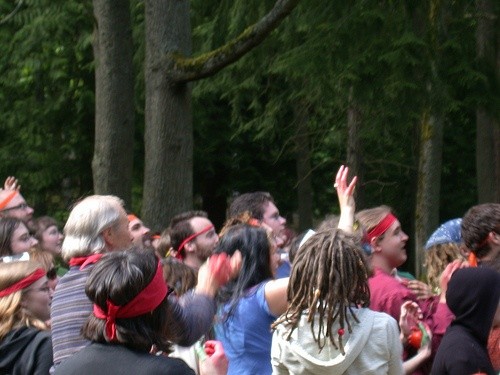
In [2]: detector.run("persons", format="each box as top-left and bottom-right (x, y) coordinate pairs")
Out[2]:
(52, 247), (228, 375)
(49, 195), (242, 375)
(0, 261), (54, 375)
(212, 165), (359, 375)
(126, 192), (294, 375)
(0, 177), (70, 292)
(353, 204), (500, 375)
(271, 229), (406, 375)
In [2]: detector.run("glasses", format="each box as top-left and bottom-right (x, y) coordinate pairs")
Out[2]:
(30, 285), (51, 293)
(0, 203), (27, 212)
(47, 267), (59, 279)
(11, 233), (33, 243)
